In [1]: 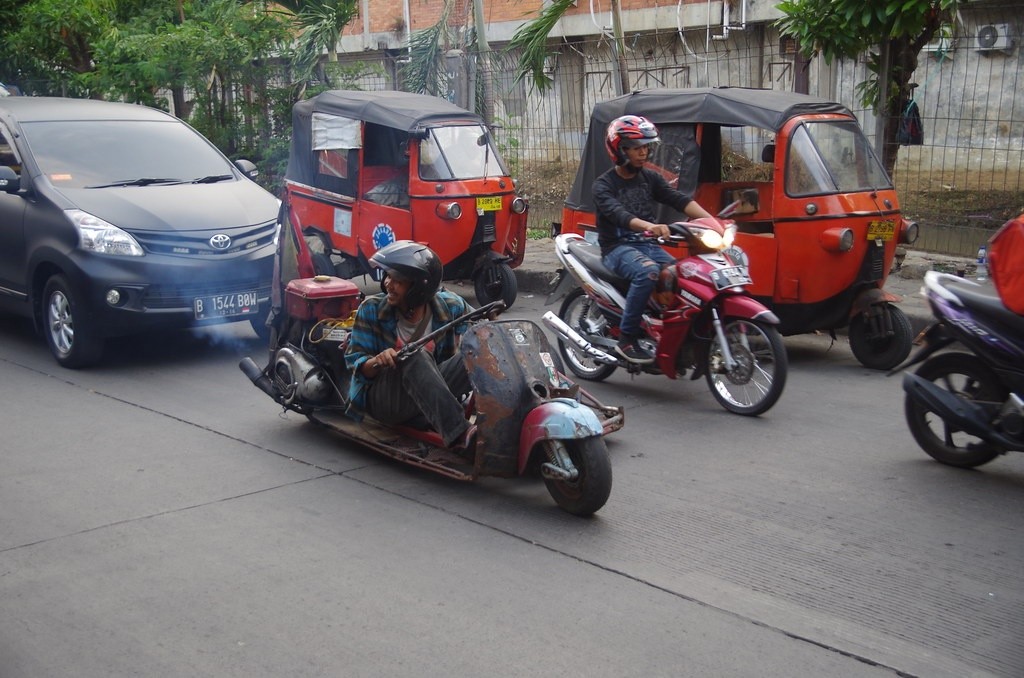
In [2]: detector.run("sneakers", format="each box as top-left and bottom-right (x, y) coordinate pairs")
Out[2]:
(614, 339), (653, 362)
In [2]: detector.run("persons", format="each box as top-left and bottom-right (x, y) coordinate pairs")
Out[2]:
(593, 115), (725, 363)
(345, 239), (485, 450)
(428, 130), (490, 178)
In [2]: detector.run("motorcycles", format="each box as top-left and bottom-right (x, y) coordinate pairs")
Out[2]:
(560, 87), (919, 370)
(542, 219), (787, 416)
(282, 89), (527, 315)
(886, 270), (1024, 468)
(239, 273), (624, 514)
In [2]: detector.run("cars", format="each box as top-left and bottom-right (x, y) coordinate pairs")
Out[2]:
(0, 96), (285, 369)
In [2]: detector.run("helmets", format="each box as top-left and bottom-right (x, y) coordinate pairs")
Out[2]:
(605, 115), (659, 165)
(369, 239), (443, 306)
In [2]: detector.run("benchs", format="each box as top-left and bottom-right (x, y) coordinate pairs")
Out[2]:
(697, 181), (774, 233)
(362, 164), (427, 209)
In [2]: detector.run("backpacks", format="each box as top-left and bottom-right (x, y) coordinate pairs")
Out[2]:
(987, 213), (1024, 314)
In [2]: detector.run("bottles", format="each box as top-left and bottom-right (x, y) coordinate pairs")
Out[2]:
(977, 246), (988, 281)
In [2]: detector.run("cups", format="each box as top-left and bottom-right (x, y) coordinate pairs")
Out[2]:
(956, 264), (966, 276)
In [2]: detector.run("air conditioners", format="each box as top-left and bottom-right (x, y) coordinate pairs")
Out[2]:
(921, 23), (955, 52)
(973, 23), (1016, 51)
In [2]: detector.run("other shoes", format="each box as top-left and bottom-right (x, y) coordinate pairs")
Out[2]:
(453, 425), (476, 451)
(563, 383), (579, 397)
